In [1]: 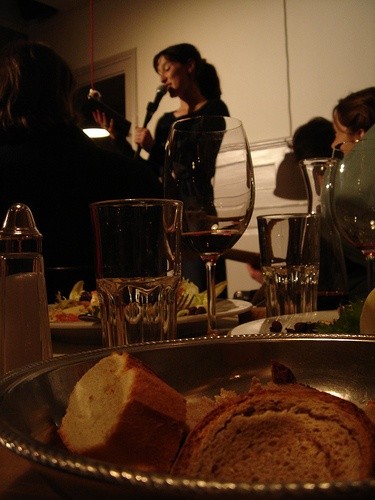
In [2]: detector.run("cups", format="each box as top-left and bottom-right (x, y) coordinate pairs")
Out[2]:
(303, 137), (375, 311)
(89, 198), (183, 347)
(257, 213), (320, 317)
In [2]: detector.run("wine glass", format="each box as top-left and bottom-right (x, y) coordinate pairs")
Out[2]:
(163, 115), (254, 336)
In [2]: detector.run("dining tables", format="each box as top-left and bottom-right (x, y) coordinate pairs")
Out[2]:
(0, 306), (375, 500)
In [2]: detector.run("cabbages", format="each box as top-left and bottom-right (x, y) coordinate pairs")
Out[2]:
(68, 279), (208, 319)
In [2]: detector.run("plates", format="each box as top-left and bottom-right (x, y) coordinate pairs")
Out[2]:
(0, 333), (374, 498)
(228, 310), (340, 335)
(48, 299), (253, 339)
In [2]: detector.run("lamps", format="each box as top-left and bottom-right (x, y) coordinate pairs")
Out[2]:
(77, 0), (111, 138)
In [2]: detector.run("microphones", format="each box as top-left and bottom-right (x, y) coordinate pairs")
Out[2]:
(146, 85), (168, 122)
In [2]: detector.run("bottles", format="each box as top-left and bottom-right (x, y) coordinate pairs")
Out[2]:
(0, 203), (53, 374)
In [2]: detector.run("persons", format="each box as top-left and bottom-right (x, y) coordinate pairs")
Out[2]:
(0, 41), (163, 302)
(246, 86), (375, 318)
(93, 43), (228, 301)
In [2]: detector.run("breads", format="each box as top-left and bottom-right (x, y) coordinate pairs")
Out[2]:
(57, 348), (375, 486)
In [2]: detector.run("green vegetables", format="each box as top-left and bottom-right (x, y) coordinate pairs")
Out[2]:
(283, 297), (365, 335)
